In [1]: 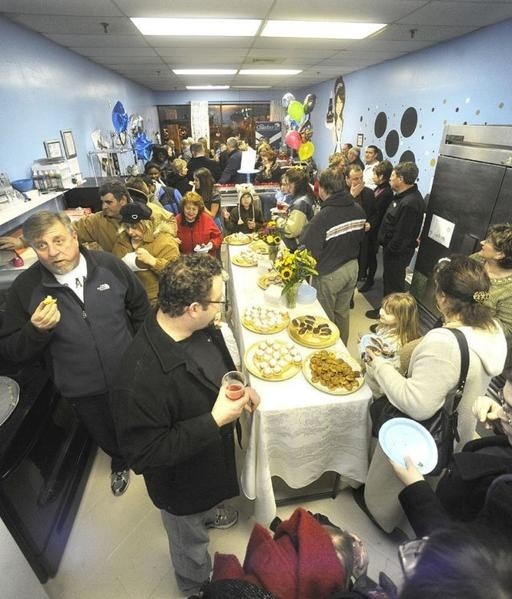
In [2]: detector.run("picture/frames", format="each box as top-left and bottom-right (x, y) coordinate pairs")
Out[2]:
(60, 130), (78, 159)
(357, 134), (363, 147)
(44, 140), (64, 160)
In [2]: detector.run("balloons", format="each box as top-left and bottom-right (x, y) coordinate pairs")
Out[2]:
(128, 111), (146, 138)
(279, 89), (318, 162)
(112, 100), (128, 134)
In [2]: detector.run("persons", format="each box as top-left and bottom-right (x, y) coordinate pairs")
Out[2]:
(351, 253), (507, 543)
(0, 211), (154, 497)
(388, 371), (512, 540)
(395, 520), (511, 598)
(107, 252), (261, 599)
(429, 219), (511, 378)
(0, 132), (428, 347)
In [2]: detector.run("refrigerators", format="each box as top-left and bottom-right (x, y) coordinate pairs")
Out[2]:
(408, 155), (511, 337)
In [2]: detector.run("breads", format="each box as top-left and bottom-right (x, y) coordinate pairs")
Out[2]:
(253, 339), (302, 378)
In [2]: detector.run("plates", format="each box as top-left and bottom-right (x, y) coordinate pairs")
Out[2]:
(378, 417), (440, 476)
(224, 233), (252, 245)
(240, 303), (290, 335)
(193, 246), (210, 252)
(302, 350), (365, 395)
(232, 252), (257, 267)
(362, 335), (396, 363)
(251, 242), (269, 255)
(243, 340), (302, 382)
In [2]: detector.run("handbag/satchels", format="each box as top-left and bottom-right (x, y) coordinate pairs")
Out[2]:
(368, 393), (460, 479)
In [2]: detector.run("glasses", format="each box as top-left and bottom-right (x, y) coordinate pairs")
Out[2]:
(414, 539), (427, 559)
(187, 299), (229, 314)
(497, 388), (512, 425)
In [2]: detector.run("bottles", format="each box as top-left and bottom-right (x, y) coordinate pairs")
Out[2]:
(33, 170), (65, 192)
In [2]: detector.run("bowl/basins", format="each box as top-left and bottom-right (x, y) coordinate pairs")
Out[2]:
(295, 286), (317, 304)
(12, 179), (33, 191)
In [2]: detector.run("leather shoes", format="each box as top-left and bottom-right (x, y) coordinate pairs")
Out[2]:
(348, 270), (381, 333)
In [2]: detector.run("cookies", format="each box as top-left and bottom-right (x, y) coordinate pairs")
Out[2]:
(311, 350), (363, 392)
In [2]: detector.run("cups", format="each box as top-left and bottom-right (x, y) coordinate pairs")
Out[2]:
(277, 199), (283, 210)
(220, 370), (246, 400)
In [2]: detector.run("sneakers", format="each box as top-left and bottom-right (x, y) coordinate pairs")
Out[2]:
(111, 470), (129, 496)
(206, 505), (238, 530)
(38, 467), (66, 507)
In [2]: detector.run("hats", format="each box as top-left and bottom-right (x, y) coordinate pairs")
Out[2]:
(118, 201), (152, 224)
(235, 183), (255, 226)
(126, 179), (149, 204)
